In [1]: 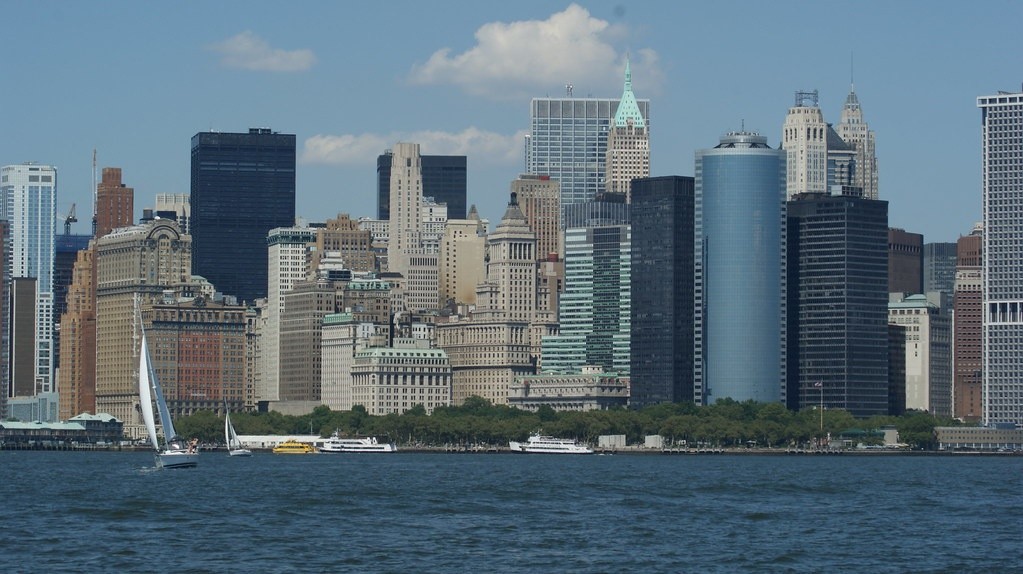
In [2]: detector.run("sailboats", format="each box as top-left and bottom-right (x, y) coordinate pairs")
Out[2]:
(224, 396), (252, 456)
(136, 300), (199, 469)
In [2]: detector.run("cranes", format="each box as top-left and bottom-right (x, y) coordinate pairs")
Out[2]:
(92, 147), (97, 235)
(64, 203), (77, 234)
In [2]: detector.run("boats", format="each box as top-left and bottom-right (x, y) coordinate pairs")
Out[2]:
(509, 428), (594, 454)
(320, 427), (398, 452)
(272, 438), (319, 454)
